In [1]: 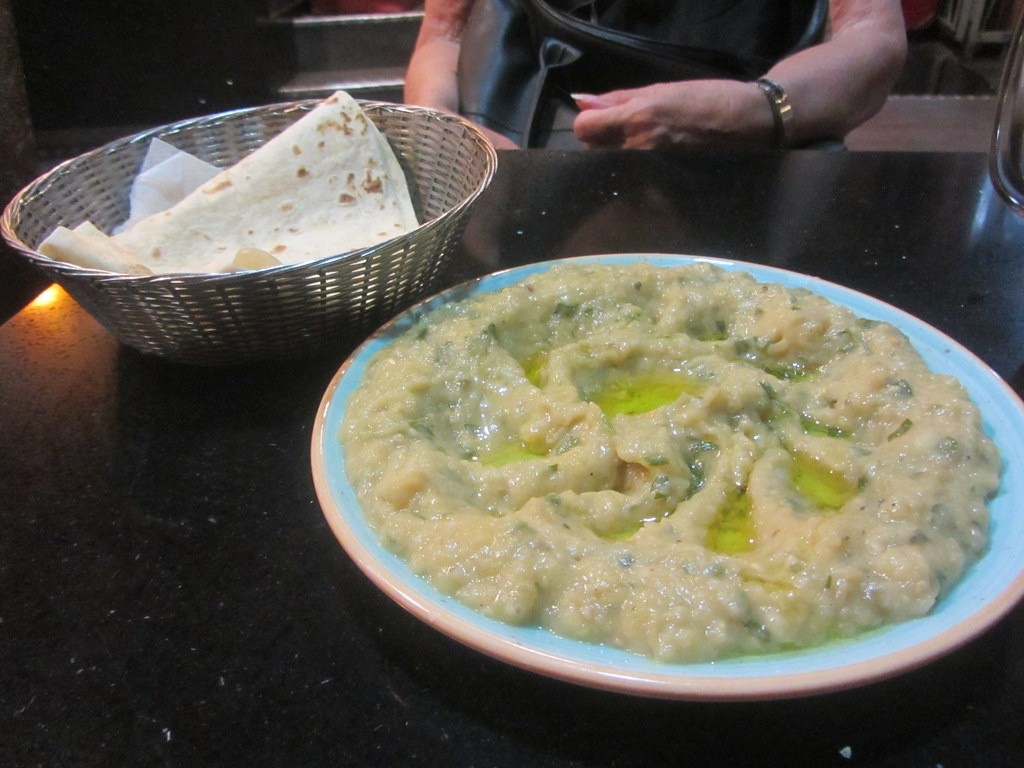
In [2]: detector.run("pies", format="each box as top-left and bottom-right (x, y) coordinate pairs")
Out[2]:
(39, 91), (419, 277)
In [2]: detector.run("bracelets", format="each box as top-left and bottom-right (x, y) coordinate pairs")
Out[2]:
(754, 76), (797, 143)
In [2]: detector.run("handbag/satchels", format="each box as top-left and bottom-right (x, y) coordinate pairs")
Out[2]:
(456, 0), (819, 144)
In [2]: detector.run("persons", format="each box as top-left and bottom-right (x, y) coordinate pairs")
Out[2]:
(401, 0), (908, 152)
(457, 151), (828, 279)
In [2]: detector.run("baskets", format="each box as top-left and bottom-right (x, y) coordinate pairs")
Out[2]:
(0, 97), (499, 364)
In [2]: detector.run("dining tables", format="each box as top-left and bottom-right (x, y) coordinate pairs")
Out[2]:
(0, 150), (1024, 768)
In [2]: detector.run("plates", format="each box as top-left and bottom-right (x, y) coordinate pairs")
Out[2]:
(309, 252), (1024, 705)
